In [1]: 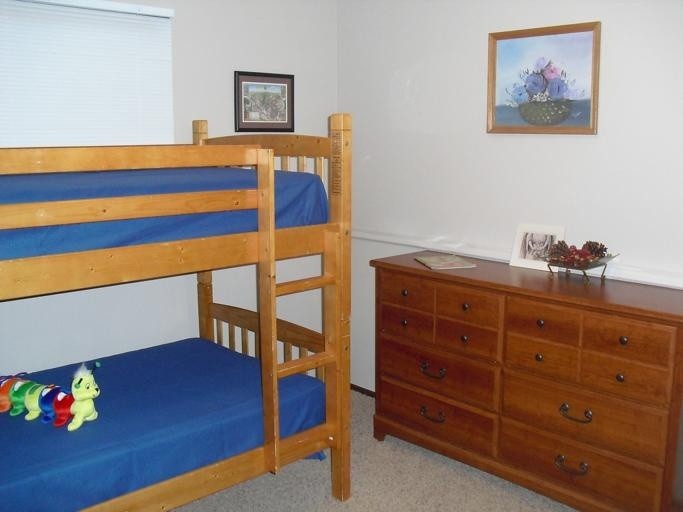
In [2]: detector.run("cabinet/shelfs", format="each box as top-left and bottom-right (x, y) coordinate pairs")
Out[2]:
(174, 188), (176, 190)
(369, 249), (683, 512)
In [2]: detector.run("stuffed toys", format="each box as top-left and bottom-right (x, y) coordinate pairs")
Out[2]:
(0, 364), (99, 430)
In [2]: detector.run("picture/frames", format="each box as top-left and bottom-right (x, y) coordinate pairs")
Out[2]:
(487, 21), (601, 134)
(509, 223), (565, 273)
(234, 71), (294, 132)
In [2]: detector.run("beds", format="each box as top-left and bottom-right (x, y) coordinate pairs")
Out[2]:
(0, 113), (351, 511)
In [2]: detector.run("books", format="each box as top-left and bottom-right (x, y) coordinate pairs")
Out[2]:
(414, 254), (477, 270)
